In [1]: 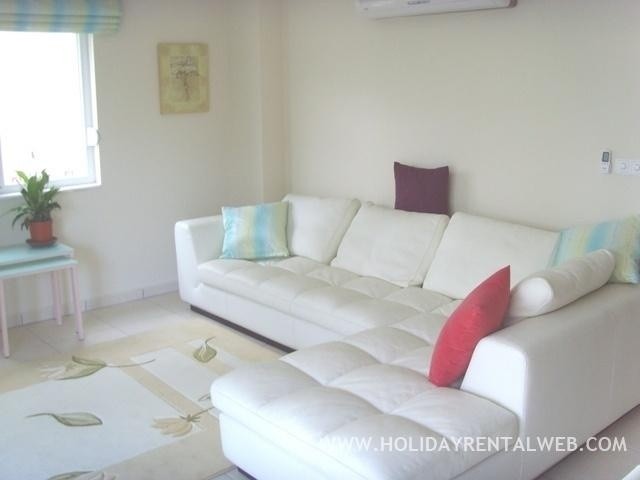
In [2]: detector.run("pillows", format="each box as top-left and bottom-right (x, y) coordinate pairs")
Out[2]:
(427, 263), (513, 388)
(219, 201), (290, 260)
(392, 158), (451, 221)
(552, 214), (640, 288)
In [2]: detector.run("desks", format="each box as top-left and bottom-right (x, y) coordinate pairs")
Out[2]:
(0, 241), (87, 358)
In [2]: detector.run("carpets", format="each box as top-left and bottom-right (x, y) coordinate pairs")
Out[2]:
(2, 317), (279, 480)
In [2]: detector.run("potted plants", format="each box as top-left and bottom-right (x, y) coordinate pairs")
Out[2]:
(0, 165), (65, 248)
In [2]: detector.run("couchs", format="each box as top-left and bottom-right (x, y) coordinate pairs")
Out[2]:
(175, 187), (640, 480)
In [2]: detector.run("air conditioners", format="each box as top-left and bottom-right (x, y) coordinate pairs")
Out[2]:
(351, 0), (519, 20)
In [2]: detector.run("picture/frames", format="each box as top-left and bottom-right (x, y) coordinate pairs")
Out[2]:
(156, 39), (212, 117)
